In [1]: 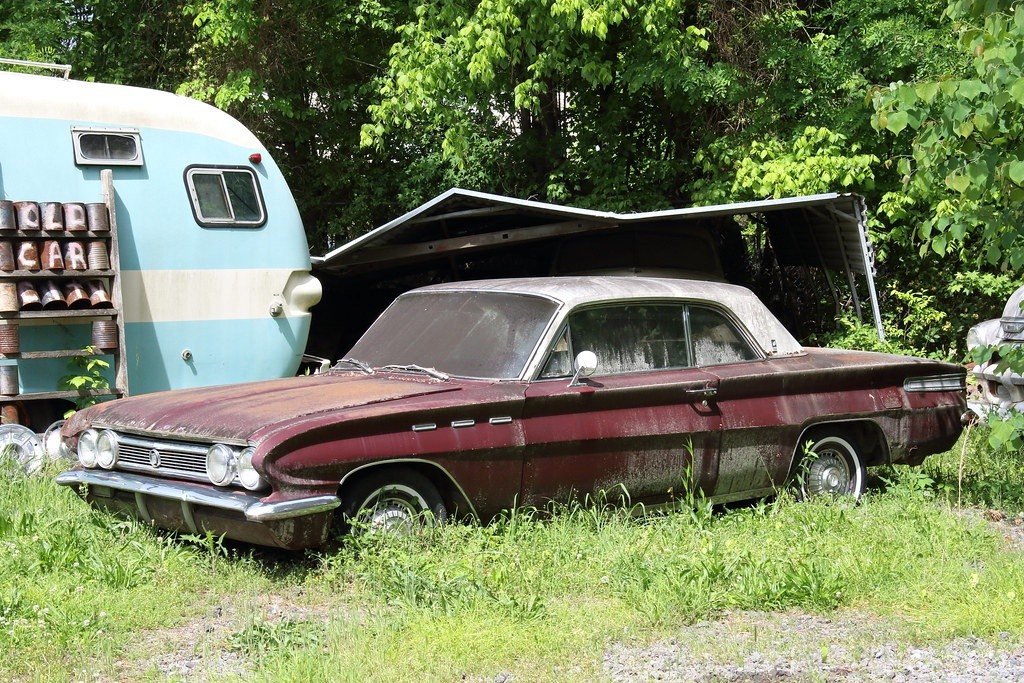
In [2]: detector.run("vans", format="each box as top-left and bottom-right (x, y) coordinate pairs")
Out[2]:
(0, 58), (323, 439)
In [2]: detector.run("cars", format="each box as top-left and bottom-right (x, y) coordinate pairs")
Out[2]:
(61, 275), (974, 557)
(966, 283), (1024, 431)
